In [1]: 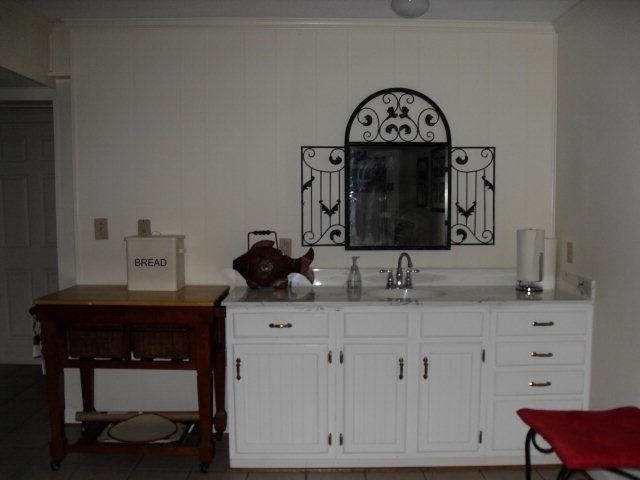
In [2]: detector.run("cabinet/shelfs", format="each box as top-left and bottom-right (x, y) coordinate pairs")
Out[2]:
(233, 308), (331, 453)
(490, 311), (588, 452)
(33, 284), (230, 473)
(340, 309), (485, 454)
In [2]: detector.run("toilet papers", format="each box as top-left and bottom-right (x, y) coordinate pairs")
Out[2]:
(516, 228), (545, 282)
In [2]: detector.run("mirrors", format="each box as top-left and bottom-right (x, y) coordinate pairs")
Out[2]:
(301, 86), (496, 251)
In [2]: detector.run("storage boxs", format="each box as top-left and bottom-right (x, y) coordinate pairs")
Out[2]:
(125, 234), (187, 292)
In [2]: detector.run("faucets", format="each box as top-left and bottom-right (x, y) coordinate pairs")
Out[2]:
(395, 252), (413, 288)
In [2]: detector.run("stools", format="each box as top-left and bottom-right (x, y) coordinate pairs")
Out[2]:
(516, 403), (639, 480)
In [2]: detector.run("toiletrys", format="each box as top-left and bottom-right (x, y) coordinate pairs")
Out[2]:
(347, 256), (363, 289)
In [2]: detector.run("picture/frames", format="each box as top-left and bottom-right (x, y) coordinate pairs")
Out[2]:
(416, 156), (429, 207)
(430, 146), (445, 213)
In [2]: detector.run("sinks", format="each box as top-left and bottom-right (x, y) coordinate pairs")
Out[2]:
(366, 287), (447, 299)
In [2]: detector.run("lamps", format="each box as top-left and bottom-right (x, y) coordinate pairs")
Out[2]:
(391, 0), (430, 19)
